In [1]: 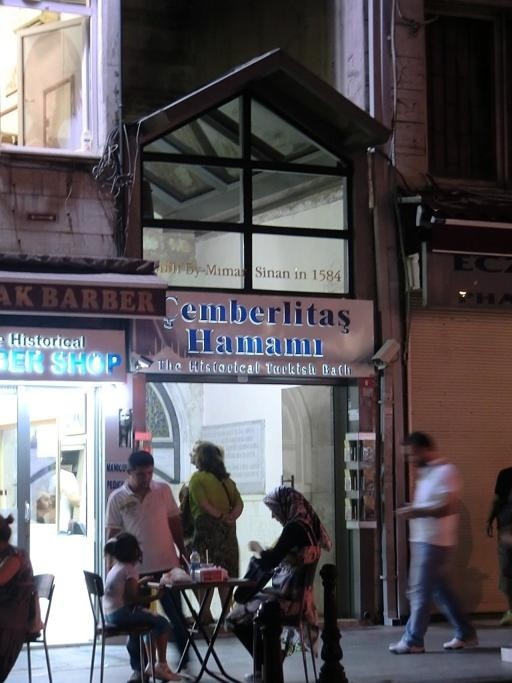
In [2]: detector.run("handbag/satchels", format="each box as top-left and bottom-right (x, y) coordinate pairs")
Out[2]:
(29, 591), (44, 634)
(235, 556), (273, 604)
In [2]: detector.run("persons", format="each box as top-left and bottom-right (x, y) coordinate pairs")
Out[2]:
(185, 441), (244, 625)
(483, 467), (512, 626)
(101, 532), (182, 681)
(0, 514), (41, 683)
(224, 485), (333, 683)
(59, 453), (82, 533)
(105, 450), (195, 683)
(387, 431), (479, 654)
(188, 439), (215, 623)
(36, 491), (53, 524)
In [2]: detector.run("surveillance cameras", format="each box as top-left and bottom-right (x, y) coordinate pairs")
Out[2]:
(136, 356), (152, 371)
(371, 339), (401, 370)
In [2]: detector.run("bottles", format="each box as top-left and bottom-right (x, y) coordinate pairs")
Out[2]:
(189, 547), (201, 582)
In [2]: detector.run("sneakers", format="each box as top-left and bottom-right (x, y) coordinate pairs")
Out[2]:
(145, 663), (180, 680)
(389, 642), (425, 653)
(190, 611), (235, 637)
(245, 672), (261, 682)
(444, 637), (479, 649)
(501, 612), (512, 624)
(128, 671), (149, 682)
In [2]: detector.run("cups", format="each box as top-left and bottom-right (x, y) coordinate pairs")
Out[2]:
(199, 563), (214, 569)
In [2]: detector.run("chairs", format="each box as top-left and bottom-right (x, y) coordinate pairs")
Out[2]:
(22, 574), (54, 683)
(244, 560), (318, 683)
(83, 571), (156, 683)
